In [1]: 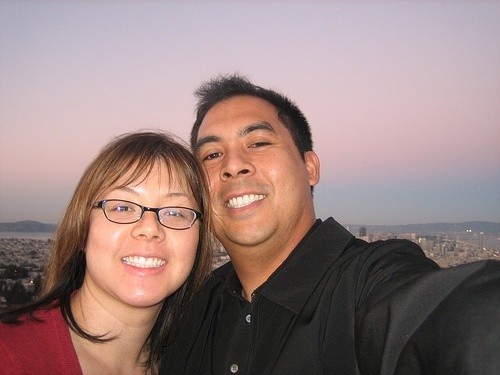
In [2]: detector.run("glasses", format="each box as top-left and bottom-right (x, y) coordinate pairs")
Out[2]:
(92, 199), (203, 230)
